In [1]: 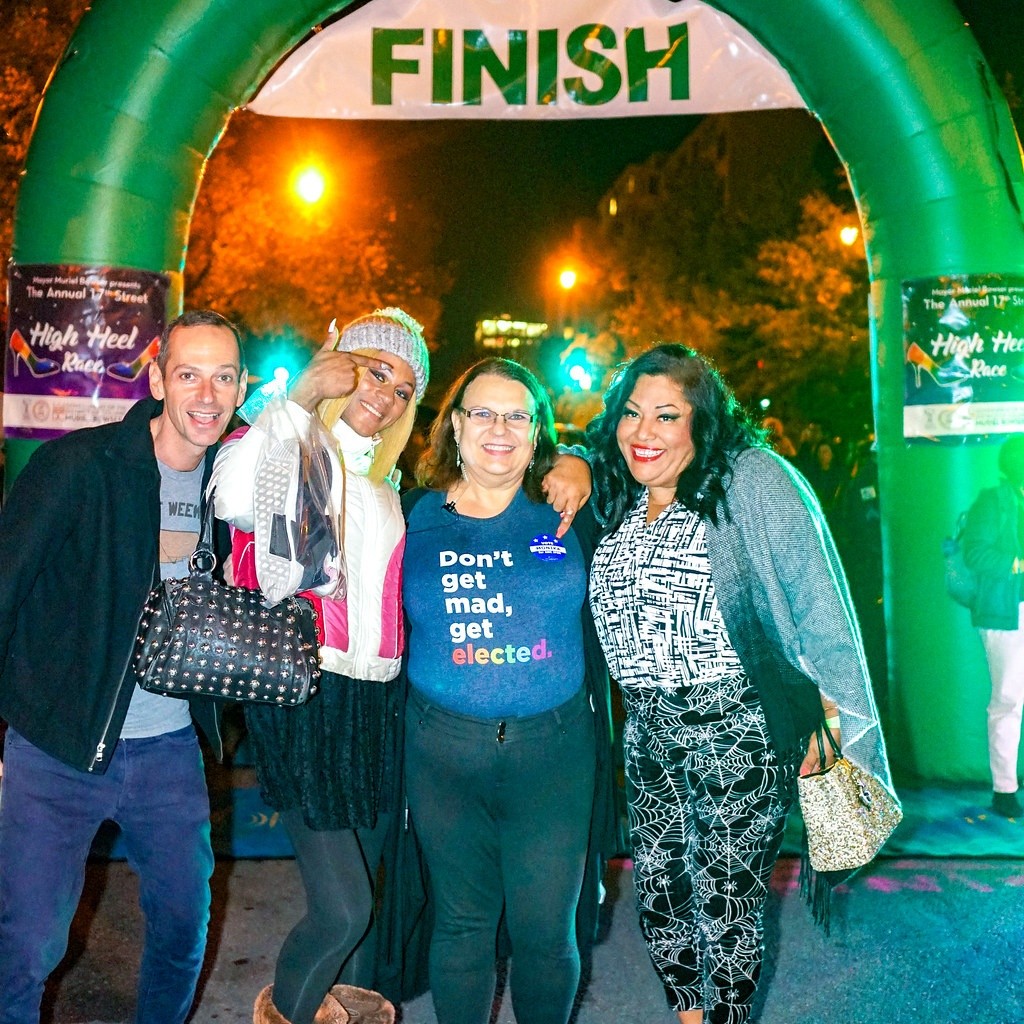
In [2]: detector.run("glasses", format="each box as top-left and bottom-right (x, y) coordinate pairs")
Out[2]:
(455, 403), (538, 429)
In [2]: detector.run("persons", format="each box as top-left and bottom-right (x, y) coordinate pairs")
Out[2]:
(963, 436), (1024, 817)
(541, 342), (844, 1024)
(0, 308), (247, 1024)
(399, 355), (615, 1024)
(204, 304), (430, 1024)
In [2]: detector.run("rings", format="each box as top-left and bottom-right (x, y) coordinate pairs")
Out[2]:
(565, 511), (575, 518)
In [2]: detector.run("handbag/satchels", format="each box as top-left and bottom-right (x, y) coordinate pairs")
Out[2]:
(131, 487), (321, 706)
(940, 490), (1001, 605)
(797, 719), (902, 871)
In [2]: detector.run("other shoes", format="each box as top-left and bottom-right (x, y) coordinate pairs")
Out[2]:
(991, 792), (1024, 817)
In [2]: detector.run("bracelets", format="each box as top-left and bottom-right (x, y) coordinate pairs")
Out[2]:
(823, 707), (838, 712)
(826, 716), (840, 728)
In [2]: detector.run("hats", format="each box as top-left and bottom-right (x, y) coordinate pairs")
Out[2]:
(333, 306), (430, 402)
